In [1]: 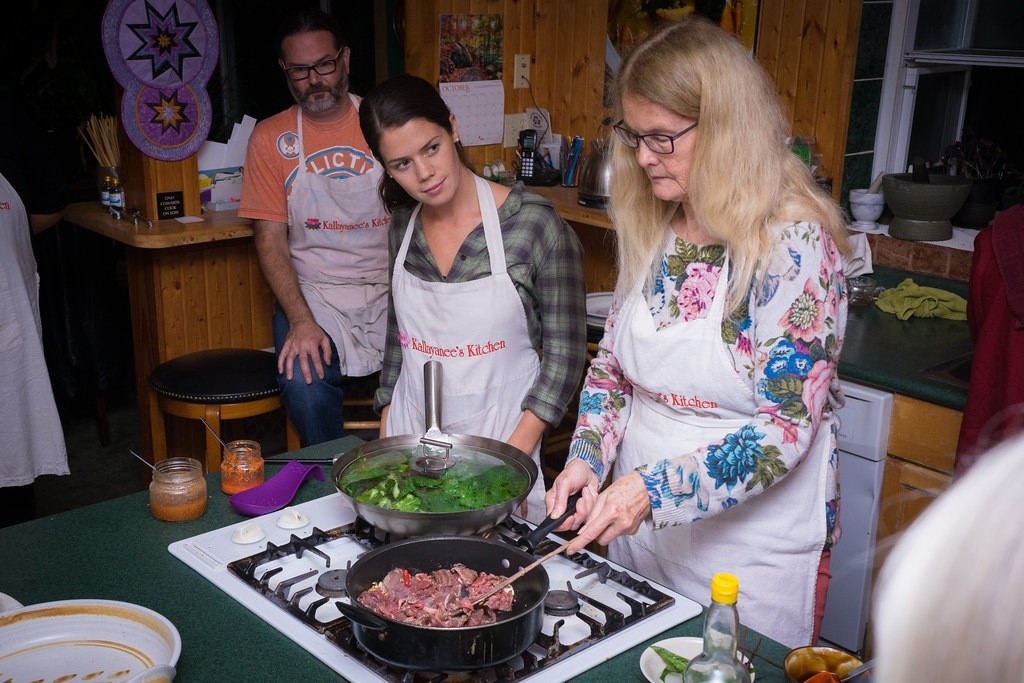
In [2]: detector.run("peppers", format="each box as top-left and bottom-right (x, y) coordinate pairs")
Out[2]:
(404, 570), (409, 586)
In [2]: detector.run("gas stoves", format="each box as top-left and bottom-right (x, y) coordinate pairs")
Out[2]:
(168, 492), (703, 683)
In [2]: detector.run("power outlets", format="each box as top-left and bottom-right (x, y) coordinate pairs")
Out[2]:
(514, 56), (532, 89)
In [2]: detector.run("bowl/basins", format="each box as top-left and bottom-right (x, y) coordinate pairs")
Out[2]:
(639, 636), (756, 683)
(785, 646), (872, 683)
(585, 291), (613, 318)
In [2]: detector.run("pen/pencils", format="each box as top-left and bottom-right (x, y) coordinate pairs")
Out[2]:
(561, 136), (584, 186)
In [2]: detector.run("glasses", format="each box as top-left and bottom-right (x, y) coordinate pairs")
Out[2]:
(280, 48), (344, 82)
(613, 116), (698, 154)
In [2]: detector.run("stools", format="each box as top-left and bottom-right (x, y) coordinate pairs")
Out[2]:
(286, 372), (396, 451)
(152, 347), (286, 476)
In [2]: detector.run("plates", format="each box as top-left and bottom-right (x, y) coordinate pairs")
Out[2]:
(0, 599), (181, 683)
(587, 316), (606, 326)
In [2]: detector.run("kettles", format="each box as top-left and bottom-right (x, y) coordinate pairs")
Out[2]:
(578, 140), (614, 209)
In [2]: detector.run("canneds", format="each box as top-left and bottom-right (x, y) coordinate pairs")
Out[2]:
(149, 456), (206, 522)
(220, 440), (264, 495)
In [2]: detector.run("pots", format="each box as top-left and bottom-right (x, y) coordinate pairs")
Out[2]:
(331, 361), (539, 536)
(335, 489), (584, 671)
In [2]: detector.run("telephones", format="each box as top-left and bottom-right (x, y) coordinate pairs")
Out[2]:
(517, 129), (561, 187)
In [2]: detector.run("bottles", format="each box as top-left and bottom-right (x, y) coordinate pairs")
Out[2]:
(149, 457), (206, 521)
(101, 175), (125, 211)
(683, 574), (752, 683)
(221, 439), (265, 495)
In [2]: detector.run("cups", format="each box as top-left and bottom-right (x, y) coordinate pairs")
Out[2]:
(845, 276), (887, 305)
(559, 150), (582, 188)
(96, 166), (123, 204)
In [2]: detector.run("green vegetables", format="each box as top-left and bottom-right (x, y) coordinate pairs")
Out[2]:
(650, 645), (755, 683)
(339, 450), (518, 513)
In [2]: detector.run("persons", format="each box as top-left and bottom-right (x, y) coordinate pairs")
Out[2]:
(955, 203), (1023, 480)
(238, 13), (394, 447)
(360, 75), (586, 522)
(545, 18), (850, 648)
(0, 173), (71, 526)
(870, 429), (1024, 683)
